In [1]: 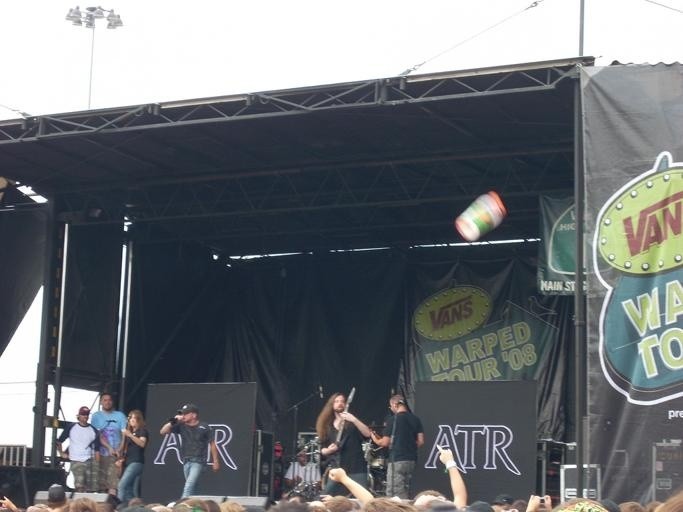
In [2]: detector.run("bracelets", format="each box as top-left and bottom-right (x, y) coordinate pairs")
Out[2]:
(167, 418), (177, 426)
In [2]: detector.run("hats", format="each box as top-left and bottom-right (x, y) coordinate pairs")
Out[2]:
(79, 407), (91, 416)
(49, 483), (65, 501)
(177, 403), (200, 415)
(494, 494), (513, 504)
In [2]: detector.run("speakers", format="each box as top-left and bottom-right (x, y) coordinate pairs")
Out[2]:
(225, 495), (276, 512)
(0, 464), (65, 508)
(188, 494), (225, 506)
(537, 439), (567, 506)
(72, 492), (123, 507)
(33, 489), (72, 507)
(652, 442), (683, 502)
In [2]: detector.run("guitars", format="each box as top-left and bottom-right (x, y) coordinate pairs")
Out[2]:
(322, 389), (355, 468)
(117, 417), (131, 478)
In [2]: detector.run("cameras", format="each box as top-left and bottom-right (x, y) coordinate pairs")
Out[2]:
(540, 497), (545, 505)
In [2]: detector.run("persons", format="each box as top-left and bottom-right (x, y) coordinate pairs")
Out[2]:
(159, 402), (218, 500)
(116, 409), (148, 501)
(369, 394), (425, 500)
(2, 445), (682, 510)
(313, 392), (371, 498)
(90, 392), (127, 495)
(56, 406), (101, 493)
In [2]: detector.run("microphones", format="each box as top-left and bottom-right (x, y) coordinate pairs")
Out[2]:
(319, 384), (324, 398)
(105, 420), (118, 423)
